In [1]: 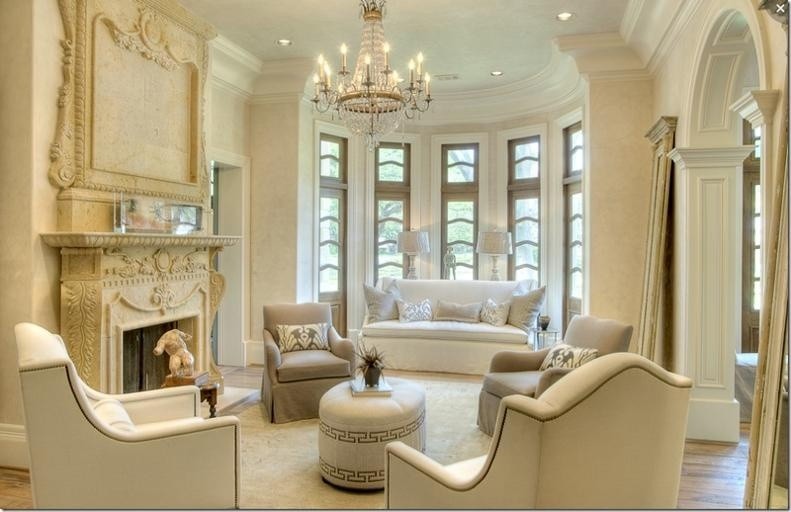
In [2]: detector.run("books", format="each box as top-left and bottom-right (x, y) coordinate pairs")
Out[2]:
(350, 380), (394, 396)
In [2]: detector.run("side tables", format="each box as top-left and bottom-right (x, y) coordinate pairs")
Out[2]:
(533, 327), (560, 351)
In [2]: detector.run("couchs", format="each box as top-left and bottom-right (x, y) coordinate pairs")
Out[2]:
(260, 302), (357, 424)
(14, 322), (242, 511)
(476, 314), (633, 440)
(355, 278), (548, 376)
(384, 351), (693, 511)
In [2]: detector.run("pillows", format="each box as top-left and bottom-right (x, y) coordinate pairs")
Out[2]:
(538, 341), (599, 373)
(434, 297), (483, 322)
(481, 296), (511, 326)
(508, 279), (546, 332)
(276, 322), (332, 354)
(395, 296), (434, 322)
(363, 279), (403, 324)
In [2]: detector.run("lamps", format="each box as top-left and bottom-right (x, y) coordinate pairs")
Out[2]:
(397, 225), (430, 279)
(476, 226), (513, 280)
(311, 1), (433, 154)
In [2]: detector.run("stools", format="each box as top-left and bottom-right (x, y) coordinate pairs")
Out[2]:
(317, 375), (427, 491)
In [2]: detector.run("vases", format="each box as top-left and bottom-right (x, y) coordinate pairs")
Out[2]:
(538, 316), (551, 330)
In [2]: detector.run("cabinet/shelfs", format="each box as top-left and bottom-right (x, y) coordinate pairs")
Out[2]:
(39, 229), (244, 397)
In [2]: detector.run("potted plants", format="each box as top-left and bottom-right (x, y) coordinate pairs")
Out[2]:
(352, 334), (392, 386)
